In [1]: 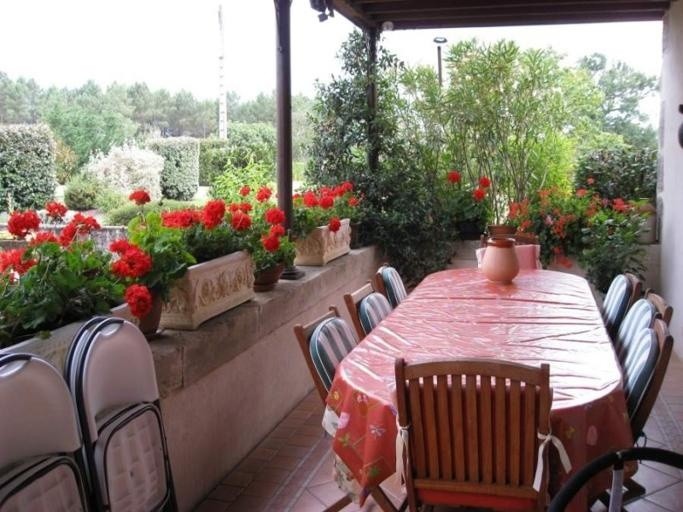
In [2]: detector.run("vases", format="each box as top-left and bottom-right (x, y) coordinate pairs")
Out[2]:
(0, 295), (143, 378)
(483, 237), (520, 285)
(635, 197), (657, 245)
(292, 217), (352, 266)
(253, 258), (283, 292)
(158, 247), (257, 331)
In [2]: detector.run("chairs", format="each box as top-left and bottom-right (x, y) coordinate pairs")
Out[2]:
(611, 287), (673, 361)
(374, 262), (407, 307)
(599, 272), (643, 340)
(291, 304), (400, 511)
(343, 277), (392, 340)
(0, 315), (178, 511)
(620, 318), (674, 444)
(388, 356), (553, 511)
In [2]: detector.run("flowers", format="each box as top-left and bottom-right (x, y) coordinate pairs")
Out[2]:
(122, 183), (285, 255)
(442, 168), (640, 268)
(0, 200), (156, 322)
(290, 181), (366, 238)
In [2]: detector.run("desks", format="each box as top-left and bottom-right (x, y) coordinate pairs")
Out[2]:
(318, 269), (638, 511)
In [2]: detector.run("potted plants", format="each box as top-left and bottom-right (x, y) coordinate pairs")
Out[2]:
(127, 210), (187, 339)
